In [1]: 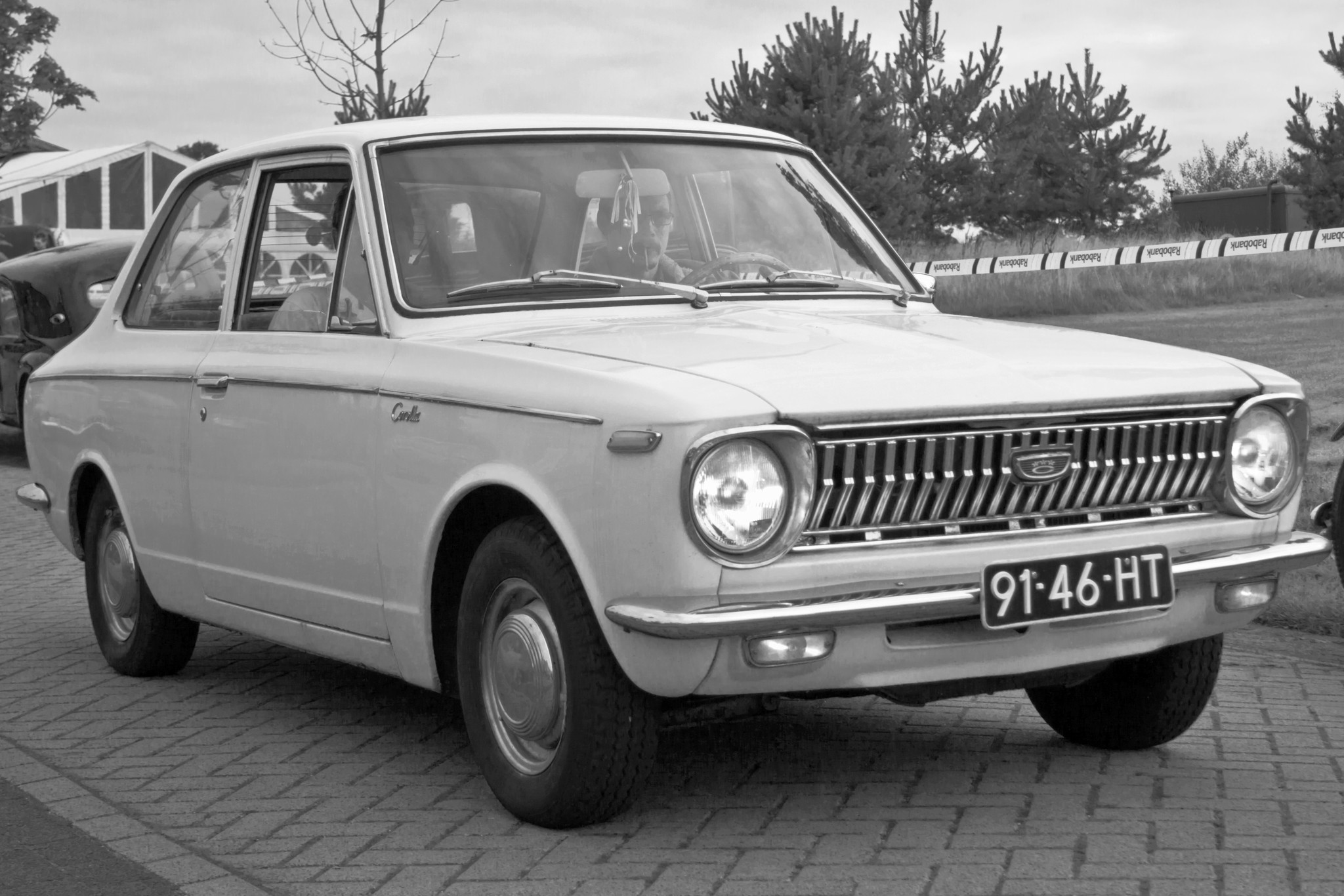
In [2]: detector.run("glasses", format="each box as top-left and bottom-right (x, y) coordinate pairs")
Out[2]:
(606, 208), (674, 226)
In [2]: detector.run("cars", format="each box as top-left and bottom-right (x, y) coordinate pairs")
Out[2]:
(0, 234), (228, 432)
(19, 118), (1331, 832)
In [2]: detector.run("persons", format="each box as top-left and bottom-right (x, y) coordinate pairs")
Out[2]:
(581, 188), (739, 286)
(265, 179), (430, 330)
